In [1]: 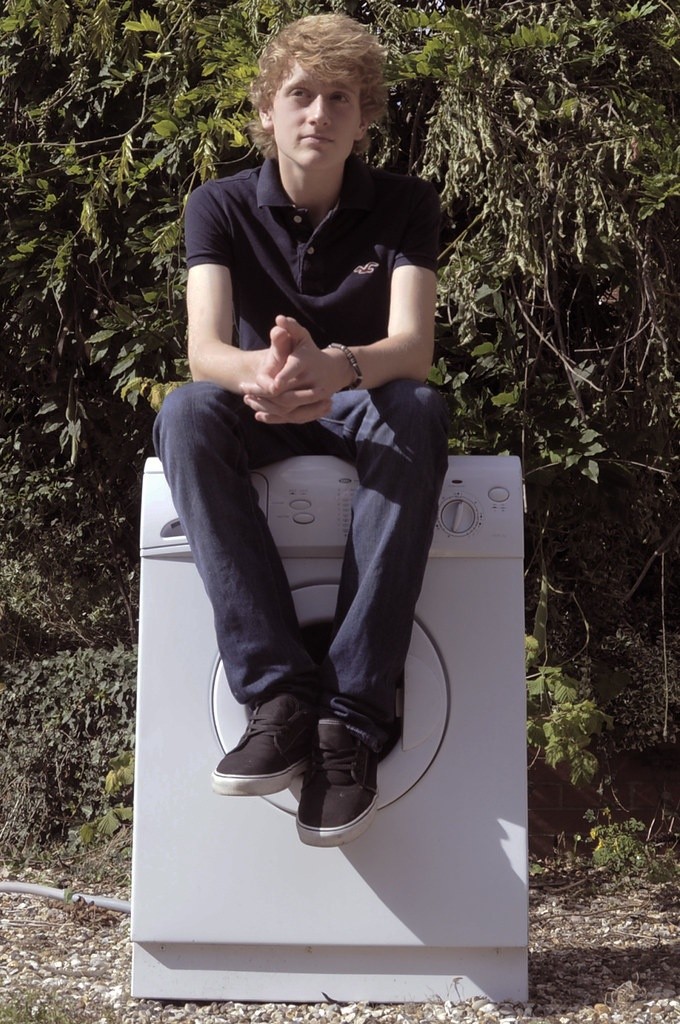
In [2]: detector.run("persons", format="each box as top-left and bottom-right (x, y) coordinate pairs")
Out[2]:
(150, 11), (450, 849)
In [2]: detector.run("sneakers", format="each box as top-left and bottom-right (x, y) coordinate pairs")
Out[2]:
(211, 694), (307, 796)
(296, 718), (381, 847)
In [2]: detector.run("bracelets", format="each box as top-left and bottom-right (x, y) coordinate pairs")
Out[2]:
(328, 342), (363, 394)
